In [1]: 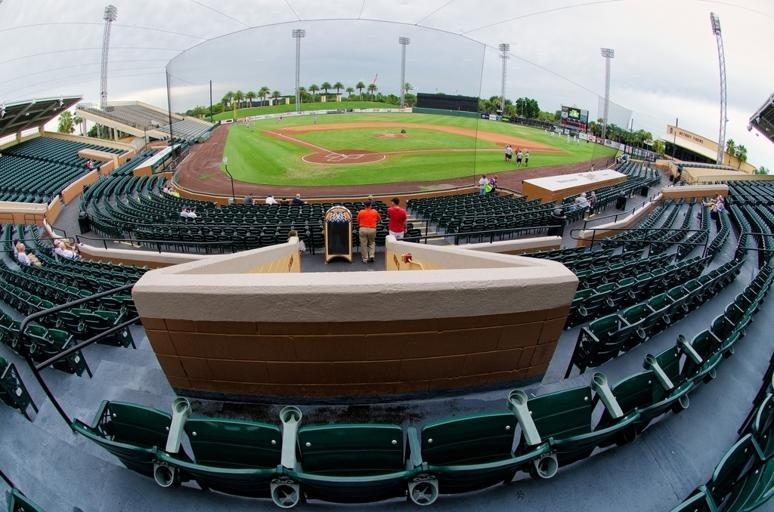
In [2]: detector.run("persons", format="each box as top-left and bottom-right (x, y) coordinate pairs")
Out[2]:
(387, 197), (407, 241)
(243, 192), (256, 207)
(188, 207), (197, 218)
(478, 174), (488, 195)
(356, 200), (381, 264)
(489, 175), (498, 192)
(701, 194), (724, 214)
(362, 195), (378, 207)
(590, 163), (595, 172)
(265, 194), (278, 205)
(568, 191), (597, 222)
(279, 196), (290, 207)
(12, 239), (34, 260)
(286, 230), (299, 246)
(15, 243), (38, 266)
(524, 151), (529, 167)
(516, 150), (523, 169)
(617, 151), (629, 165)
(53, 238), (83, 262)
(504, 145), (508, 162)
(507, 145), (512, 163)
(85, 159), (93, 170)
(292, 194), (308, 205)
(180, 207), (188, 219)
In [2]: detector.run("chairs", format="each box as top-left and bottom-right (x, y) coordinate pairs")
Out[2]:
(188, 192), (521, 511)
(521, 147), (773, 511)
(0, 138), (188, 512)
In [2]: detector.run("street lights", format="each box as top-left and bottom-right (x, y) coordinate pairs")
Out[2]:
(599, 48), (614, 139)
(292, 30), (306, 114)
(498, 43), (510, 111)
(399, 37), (409, 108)
(100, 4), (119, 108)
(710, 12), (727, 164)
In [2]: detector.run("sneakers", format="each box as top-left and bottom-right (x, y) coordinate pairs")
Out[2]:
(361, 256), (374, 262)
